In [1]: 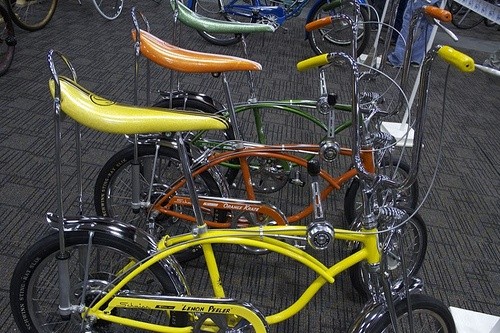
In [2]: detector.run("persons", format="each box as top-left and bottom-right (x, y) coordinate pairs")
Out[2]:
(386, 0), (440, 68)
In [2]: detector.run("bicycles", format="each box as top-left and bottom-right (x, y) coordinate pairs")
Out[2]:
(0, 0), (500, 333)
